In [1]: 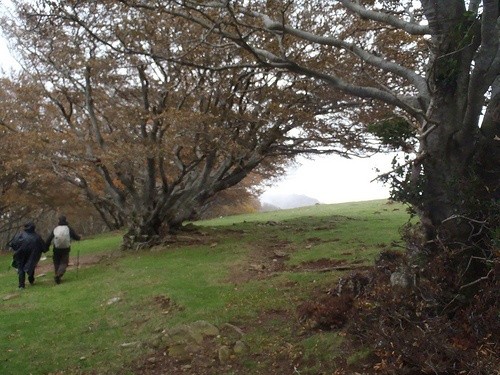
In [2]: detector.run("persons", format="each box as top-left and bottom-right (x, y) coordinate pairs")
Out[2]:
(10, 220), (49, 290)
(44, 215), (83, 284)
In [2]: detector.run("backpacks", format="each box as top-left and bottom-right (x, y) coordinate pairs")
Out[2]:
(52, 224), (71, 248)
